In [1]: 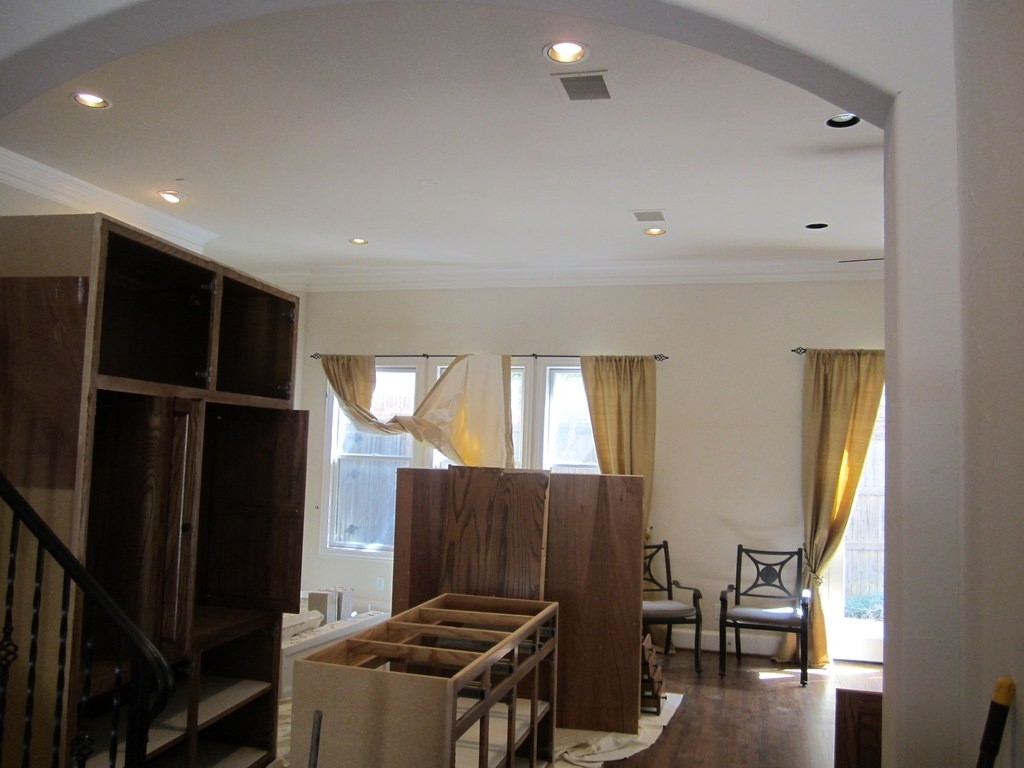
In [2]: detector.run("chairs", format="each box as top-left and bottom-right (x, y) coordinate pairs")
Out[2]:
(642, 541), (703, 674)
(718, 543), (813, 687)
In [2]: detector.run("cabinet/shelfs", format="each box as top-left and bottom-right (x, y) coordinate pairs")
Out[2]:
(0, 212), (310, 768)
(290, 592), (560, 768)
